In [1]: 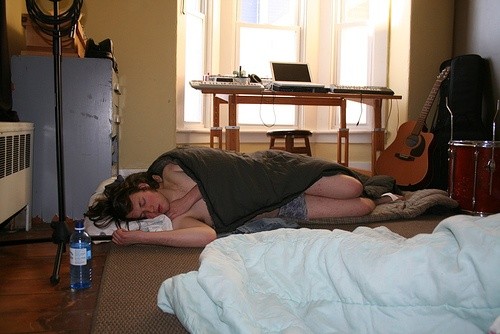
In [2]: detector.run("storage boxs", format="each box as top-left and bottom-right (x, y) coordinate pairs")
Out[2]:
(21, 13), (88, 58)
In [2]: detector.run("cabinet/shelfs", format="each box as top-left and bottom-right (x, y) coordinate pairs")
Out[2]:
(10, 55), (121, 221)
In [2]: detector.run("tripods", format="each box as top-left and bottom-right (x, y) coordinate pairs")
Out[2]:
(0, 0), (109, 282)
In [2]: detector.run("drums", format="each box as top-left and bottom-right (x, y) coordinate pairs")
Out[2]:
(447, 141), (500, 217)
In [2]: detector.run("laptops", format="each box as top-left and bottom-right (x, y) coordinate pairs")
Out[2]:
(270, 61), (325, 87)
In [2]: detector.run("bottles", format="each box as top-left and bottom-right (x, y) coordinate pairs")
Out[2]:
(69, 221), (93, 290)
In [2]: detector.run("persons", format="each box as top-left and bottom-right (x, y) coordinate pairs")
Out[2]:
(85, 160), (403, 248)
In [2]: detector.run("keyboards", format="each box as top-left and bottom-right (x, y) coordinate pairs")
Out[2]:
(189, 80), (265, 89)
(330, 84), (394, 95)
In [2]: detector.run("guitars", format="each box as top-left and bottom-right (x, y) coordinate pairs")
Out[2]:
(375, 66), (450, 186)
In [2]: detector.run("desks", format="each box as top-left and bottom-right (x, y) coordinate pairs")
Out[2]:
(200, 87), (403, 176)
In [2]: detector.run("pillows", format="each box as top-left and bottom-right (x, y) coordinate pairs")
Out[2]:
(82, 177), (172, 244)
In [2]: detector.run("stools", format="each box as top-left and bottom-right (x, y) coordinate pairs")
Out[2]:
(266, 130), (312, 156)
